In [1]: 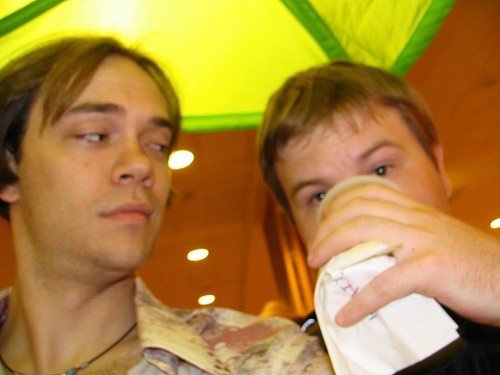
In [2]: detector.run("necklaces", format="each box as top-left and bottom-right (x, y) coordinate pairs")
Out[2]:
(1, 295), (137, 375)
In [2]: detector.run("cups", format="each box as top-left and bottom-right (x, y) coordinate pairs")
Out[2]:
(316, 177), (407, 265)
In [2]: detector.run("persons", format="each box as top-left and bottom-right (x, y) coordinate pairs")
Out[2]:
(254, 61), (500, 375)
(1, 33), (335, 374)
